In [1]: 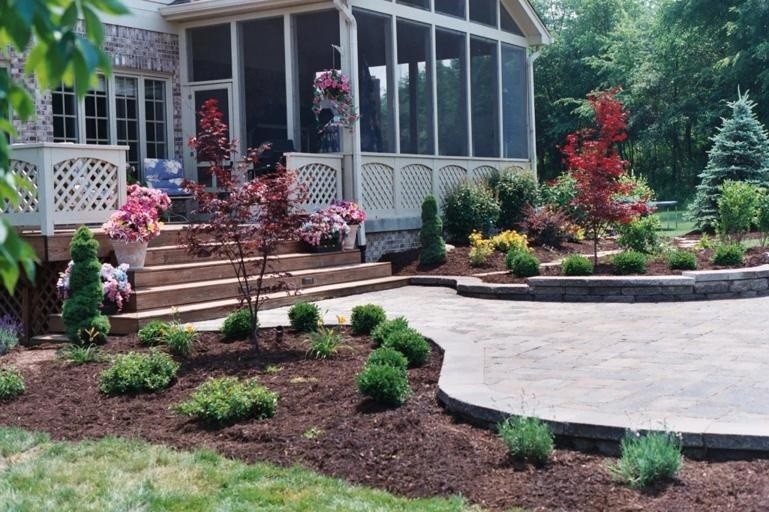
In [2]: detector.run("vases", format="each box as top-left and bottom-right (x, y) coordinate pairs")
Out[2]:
(99, 294), (118, 316)
(109, 237), (149, 269)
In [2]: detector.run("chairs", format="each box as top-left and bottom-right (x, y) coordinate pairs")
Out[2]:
(143, 158), (198, 224)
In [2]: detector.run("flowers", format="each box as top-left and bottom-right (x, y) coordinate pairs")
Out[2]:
(311, 68), (361, 135)
(288, 197), (366, 253)
(100, 182), (174, 245)
(55, 259), (133, 314)
